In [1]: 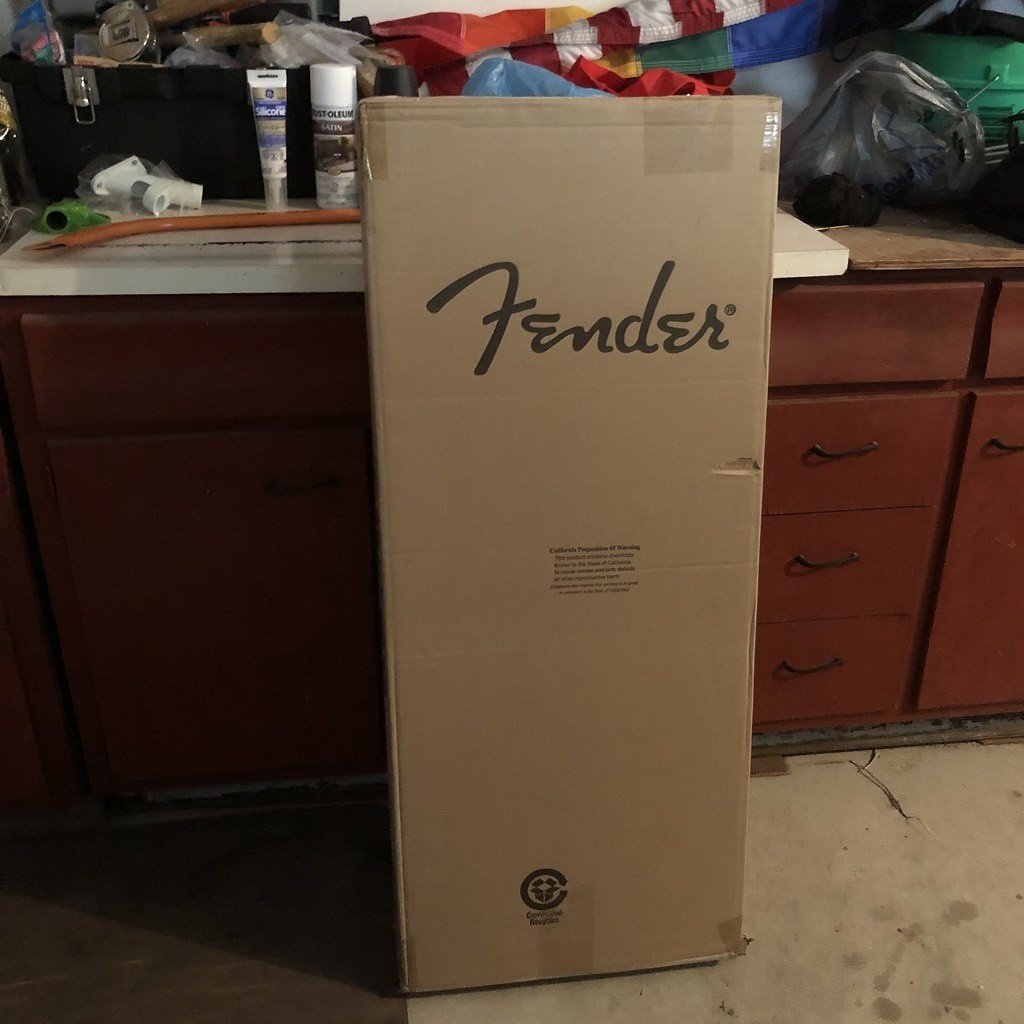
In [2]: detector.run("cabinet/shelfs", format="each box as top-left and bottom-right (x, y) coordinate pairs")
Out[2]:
(2, 211), (386, 832)
(727, 281), (977, 735)
(914, 268), (1024, 730)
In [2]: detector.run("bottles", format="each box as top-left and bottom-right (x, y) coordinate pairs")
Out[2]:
(309, 63), (361, 210)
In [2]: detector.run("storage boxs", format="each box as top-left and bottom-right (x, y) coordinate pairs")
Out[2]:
(359, 93), (784, 994)
(0, 53), (319, 212)
